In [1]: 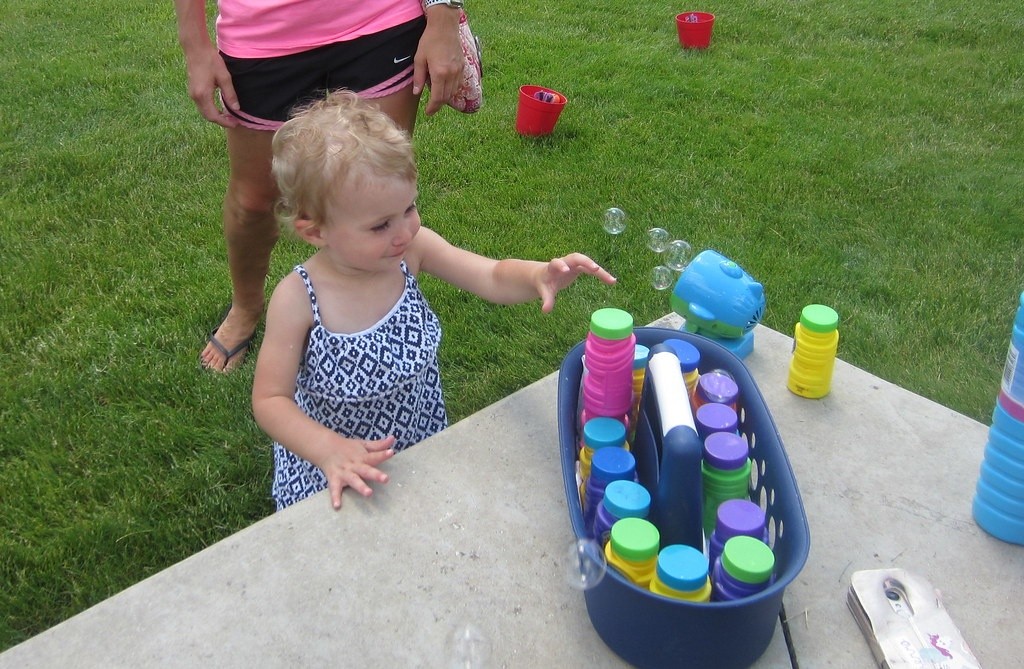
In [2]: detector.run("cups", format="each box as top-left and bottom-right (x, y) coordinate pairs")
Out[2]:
(972, 290), (1024, 547)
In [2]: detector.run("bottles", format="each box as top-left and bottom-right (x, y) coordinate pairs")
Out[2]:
(786, 304), (839, 400)
(572, 308), (777, 603)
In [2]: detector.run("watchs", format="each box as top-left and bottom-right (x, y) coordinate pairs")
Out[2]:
(424, 0), (463, 9)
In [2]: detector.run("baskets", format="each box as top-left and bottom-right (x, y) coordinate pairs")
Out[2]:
(557, 327), (810, 669)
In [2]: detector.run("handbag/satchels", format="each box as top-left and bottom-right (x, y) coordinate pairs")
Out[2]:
(425, 8), (483, 113)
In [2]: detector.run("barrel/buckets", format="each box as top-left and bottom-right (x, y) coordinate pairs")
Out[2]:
(675, 12), (715, 49)
(516, 84), (567, 138)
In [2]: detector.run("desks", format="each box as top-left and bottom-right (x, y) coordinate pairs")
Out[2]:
(0, 311), (1024, 669)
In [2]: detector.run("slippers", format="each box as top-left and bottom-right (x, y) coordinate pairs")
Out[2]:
(199, 307), (256, 374)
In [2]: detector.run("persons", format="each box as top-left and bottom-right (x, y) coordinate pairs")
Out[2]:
(252, 91), (617, 511)
(175, 0), (464, 378)
